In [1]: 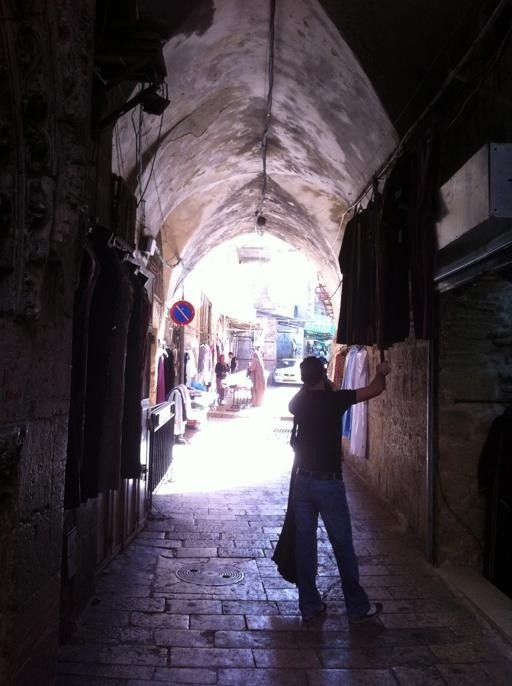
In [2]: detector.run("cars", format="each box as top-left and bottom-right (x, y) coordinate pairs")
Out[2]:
(274, 358), (304, 384)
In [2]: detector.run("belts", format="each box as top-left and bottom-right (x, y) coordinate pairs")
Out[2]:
(297, 471), (341, 479)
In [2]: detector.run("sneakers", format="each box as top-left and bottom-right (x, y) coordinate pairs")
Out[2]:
(302, 602), (328, 622)
(348, 602), (383, 624)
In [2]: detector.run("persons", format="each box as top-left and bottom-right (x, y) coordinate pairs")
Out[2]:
(285, 354), (391, 624)
(248, 352), (266, 408)
(215, 355), (231, 407)
(228, 352), (239, 373)
